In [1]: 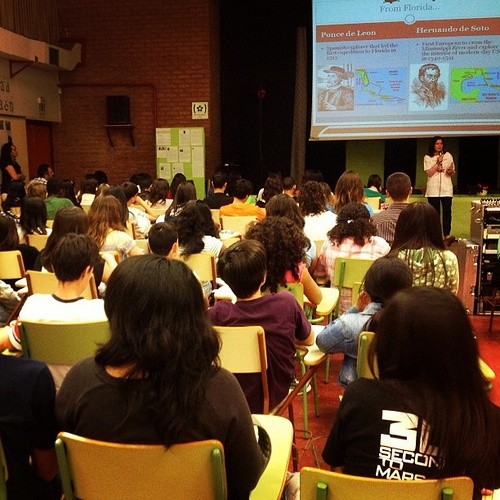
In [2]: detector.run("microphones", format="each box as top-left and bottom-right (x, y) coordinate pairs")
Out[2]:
(438, 150), (442, 165)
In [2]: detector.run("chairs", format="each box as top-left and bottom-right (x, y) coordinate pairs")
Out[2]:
(0, 197), (496, 500)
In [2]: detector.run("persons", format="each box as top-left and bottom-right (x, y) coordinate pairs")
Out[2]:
(25, 163), (54, 197)
(322, 286), (500, 500)
(87, 195), (146, 297)
(219, 169), (412, 248)
(77, 170), (233, 218)
(424, 136), (455, 239)
(204, 216), (414, 414)
(0, 234), (268, 500)
(390, 201), (459, 296)
(148, 205), (217, 309)
(0, 178), (83, 291)
(0, 143), (26, 198)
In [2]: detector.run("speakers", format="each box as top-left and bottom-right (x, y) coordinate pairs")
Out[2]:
(106, 95), (130, 124)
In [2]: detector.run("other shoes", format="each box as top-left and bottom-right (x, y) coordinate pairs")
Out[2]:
(288, 380), (312, 395)
(444, 235), (455, 246)
